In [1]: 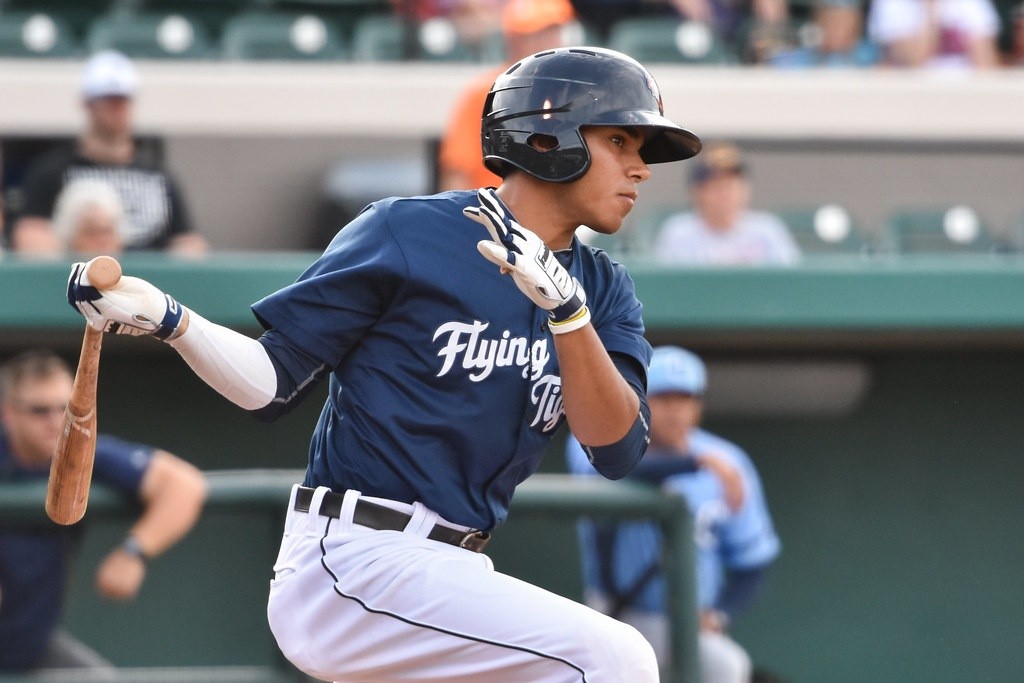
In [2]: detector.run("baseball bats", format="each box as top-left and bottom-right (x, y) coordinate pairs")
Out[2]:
(40, 253), (126, 528)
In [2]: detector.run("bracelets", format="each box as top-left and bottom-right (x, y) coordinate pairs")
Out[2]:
(548, 306), (591, 335)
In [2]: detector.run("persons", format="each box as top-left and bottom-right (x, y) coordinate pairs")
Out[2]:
(405, 0), (1024, 67)
(1, 44), (801, 683)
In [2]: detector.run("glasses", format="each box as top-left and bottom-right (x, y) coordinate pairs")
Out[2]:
(29, 403), (50, 415)
(696, 162), (745, 182)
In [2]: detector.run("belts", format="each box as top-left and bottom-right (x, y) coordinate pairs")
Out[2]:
(295, 488), (491, 553)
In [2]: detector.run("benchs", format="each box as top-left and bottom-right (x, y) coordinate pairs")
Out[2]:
(216, 10), (351, 59)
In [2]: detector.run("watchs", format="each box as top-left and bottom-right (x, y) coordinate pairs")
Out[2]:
(122, 539), (148, 565)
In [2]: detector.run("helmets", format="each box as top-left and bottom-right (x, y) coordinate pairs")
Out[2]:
(481, 46), (702, 184)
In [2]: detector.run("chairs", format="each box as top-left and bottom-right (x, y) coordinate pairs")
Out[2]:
(0, 11), (70, 56)
(884, 209), (998, 253)
(608, 17), (729, 64)
(85, 10), (209, 58)
(778, 204), (874, 256)
(355, 19), (504, 63)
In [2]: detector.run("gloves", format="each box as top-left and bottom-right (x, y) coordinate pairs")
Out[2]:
(462, 187), (586, 323)
(66, 262), (183, 340)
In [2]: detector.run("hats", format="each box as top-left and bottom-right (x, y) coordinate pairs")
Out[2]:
(502, 0), (573, 33)
(646, 345), (706, 394)
(80, 52), (136, 99)
